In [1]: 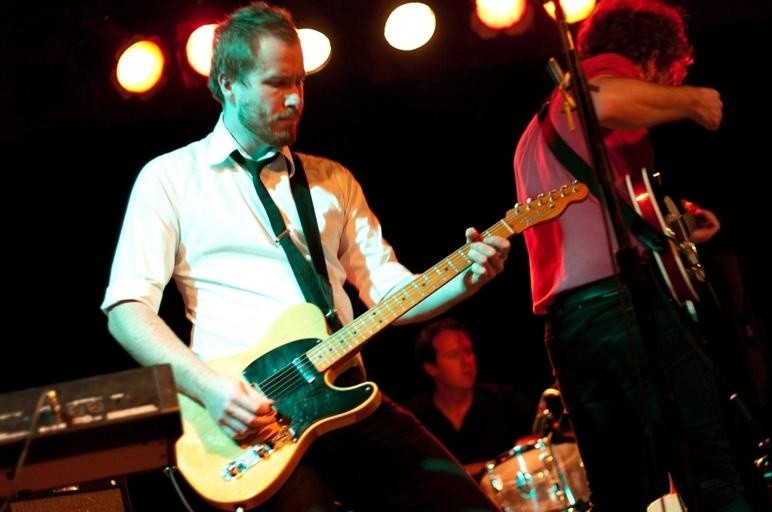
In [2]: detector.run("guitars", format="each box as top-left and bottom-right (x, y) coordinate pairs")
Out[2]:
(173, 180), (589, 511)
(623, 166), (707, 310)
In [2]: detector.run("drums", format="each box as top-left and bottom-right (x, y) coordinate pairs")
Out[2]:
(479, 441), (587, 511)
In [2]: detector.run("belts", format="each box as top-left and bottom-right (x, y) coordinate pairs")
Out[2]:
(541, 274), (639, 318)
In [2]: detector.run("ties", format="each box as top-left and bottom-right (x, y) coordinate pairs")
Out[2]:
(227, 147), (336, 320)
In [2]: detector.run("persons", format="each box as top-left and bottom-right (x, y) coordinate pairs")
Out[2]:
(101, 3), (511, 509)
(410, 322), (529, 467)
(513, 1), (770, 512)
